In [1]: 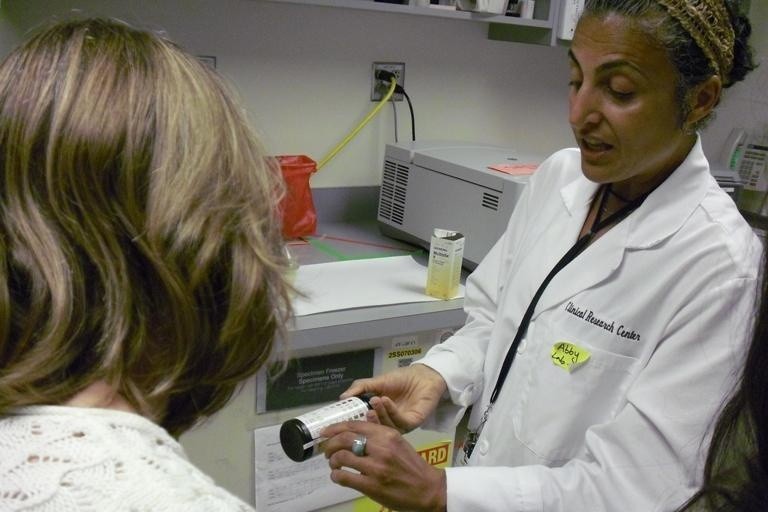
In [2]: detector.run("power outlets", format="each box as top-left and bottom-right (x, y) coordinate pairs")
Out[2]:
(371, 60), (405, 102)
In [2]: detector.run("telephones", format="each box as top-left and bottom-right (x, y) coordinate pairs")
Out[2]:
(719, 128), (767, 192)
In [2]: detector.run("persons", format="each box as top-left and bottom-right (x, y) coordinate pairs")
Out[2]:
(0, 13), (310, 512)
(313, 0), (768, 512)
(701, 228), (768, 512)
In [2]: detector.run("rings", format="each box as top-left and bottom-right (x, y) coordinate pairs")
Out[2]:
(351, 431), (369, 455)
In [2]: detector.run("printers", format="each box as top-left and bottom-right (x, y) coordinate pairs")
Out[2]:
(376, 141), (547, 276)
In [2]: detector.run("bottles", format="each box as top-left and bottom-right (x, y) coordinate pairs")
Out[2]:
(281, 392), (379, 462)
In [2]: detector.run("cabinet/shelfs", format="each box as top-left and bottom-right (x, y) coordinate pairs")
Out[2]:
(308, 0), (584, 50)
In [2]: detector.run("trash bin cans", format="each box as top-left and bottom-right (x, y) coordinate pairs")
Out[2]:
(273, 155), (318, 239)
